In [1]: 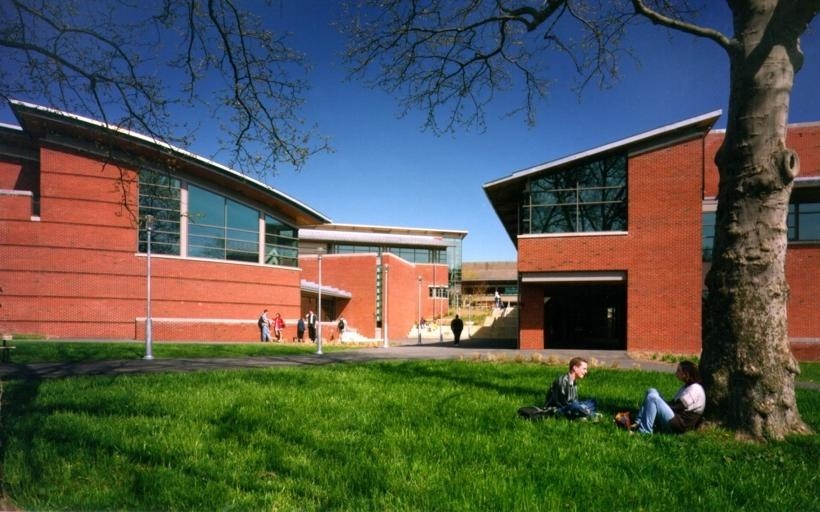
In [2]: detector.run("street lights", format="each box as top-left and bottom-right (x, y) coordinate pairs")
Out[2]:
(145, 214), (154, 359)
(467, 293), (472, 337)
(383, 263), (390, 348)
(438, 285), (445, 344)
(417, 275), (423, 346)
(316, 247), (325, 356)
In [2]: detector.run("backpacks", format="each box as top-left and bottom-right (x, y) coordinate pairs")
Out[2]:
(338, 319), (344, 329)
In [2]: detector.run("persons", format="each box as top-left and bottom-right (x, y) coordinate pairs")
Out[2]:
(258, 308), (285, 343)
(450, 314), (464, 346)
(297, 310), (317, 343)
(544, 356), (706, 437)
(494, 291), (503, 309)
(337, 315), (347, 342)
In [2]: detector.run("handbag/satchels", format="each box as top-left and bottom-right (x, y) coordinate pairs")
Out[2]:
(517, 406), (543, 417)
